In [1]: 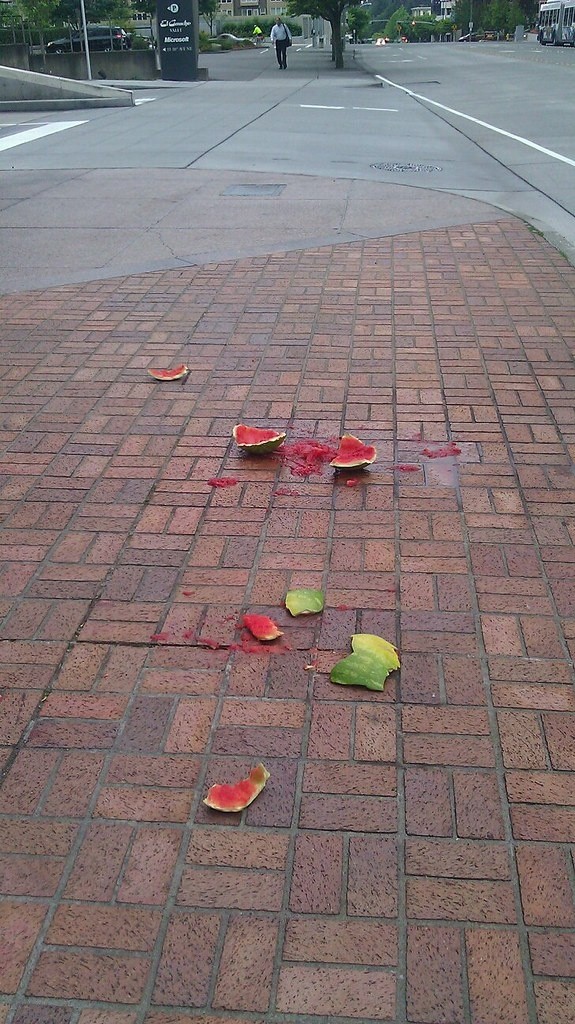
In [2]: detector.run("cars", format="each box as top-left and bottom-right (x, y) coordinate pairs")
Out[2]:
(206, 34), (251, 47)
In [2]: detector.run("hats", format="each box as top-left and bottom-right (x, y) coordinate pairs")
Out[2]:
(254, 25), (256, 27)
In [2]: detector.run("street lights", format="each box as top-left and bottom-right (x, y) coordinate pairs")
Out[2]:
(359, 2), (372, 9)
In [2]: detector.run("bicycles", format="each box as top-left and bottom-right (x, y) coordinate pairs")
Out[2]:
(253, 34), (266, 44)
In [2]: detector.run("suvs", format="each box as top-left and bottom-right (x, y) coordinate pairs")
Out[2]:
(45, 24), (132, 51)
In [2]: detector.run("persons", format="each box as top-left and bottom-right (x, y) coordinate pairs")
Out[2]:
(253, 25), (263, 38)
(270, 17), (292, 70)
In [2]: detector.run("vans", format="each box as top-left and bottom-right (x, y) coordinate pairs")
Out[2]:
(400, 36), (408, 44)
(459, 32), (479, 42)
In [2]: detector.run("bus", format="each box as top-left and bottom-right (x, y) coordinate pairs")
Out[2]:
(536, 1), (575, 47)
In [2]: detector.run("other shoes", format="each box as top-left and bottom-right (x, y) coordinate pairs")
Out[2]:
(279, 64), (282, 69)
(284, 65), (287, 69)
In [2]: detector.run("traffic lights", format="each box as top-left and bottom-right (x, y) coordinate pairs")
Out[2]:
(411, 20), (416, 26)
(399, 25), (402, 30)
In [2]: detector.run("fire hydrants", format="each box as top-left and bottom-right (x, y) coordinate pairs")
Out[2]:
(506, 34), (509, 41)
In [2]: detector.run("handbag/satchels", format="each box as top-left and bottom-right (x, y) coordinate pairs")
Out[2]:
(285, 37), (289, 47)
